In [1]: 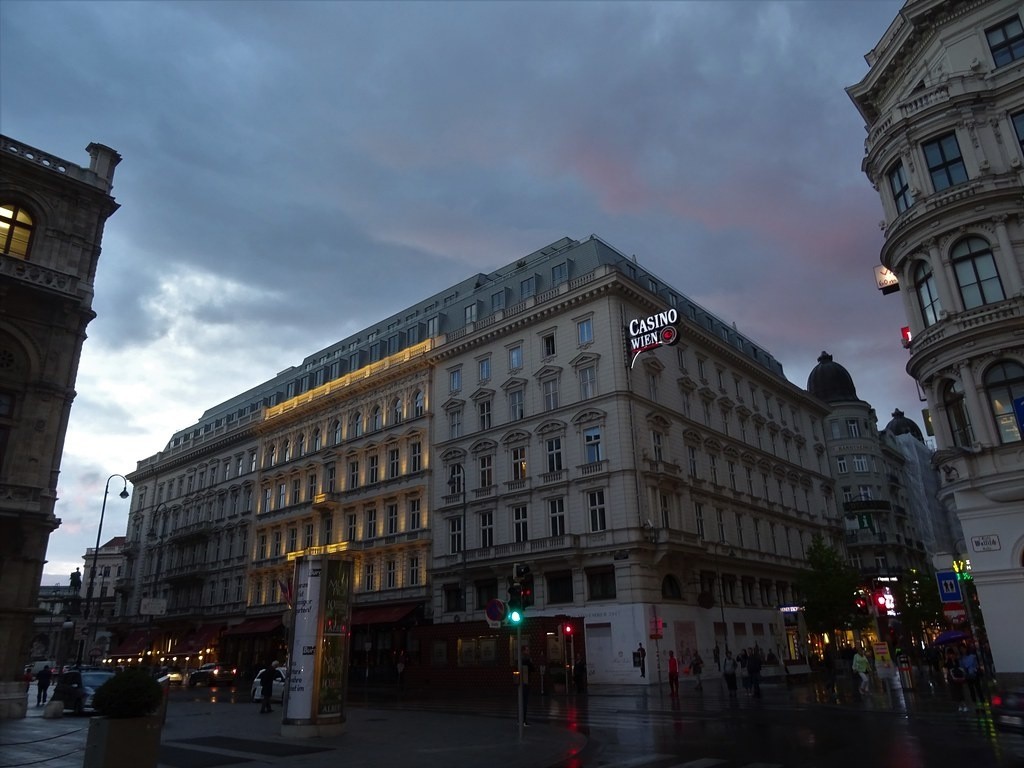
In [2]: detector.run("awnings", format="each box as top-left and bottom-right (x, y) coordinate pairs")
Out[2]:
(351, 603), (418, 621)
(109, 631), (158, 655)
(167, 623), (223, 654)
(225, 615), (282, 633)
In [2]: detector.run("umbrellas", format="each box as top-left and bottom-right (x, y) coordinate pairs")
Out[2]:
(934, 630), (967, 644)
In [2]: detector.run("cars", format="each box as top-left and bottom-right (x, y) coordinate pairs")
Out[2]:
(250, 667), (287, 707)
(992, 690), (1024, 734)
(53, 670), (115, 717)
(188, 662), (239, 686)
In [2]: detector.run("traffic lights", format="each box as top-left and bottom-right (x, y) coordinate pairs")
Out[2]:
(507, 586), (522, 624)
(565, 626), (572, 643)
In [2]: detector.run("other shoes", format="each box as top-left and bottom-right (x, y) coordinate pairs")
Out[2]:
(672, 693), (678, 697)
(859, 687), (865, 694)
(864, 690), (872, 695)
(959, 706), (963, 712)
(669, 692), (674, 697)
(964, 707), (969, 712)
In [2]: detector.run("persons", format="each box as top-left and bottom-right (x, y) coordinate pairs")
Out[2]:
(25, 668), (35, 692)
(689, 648), (705, 689)
(667, 650), (679, 696)
(36, 665), (52, 706)
(637, 642), (647, 678)
(724, 643), (978, 712)
(260, 660), (284, 713)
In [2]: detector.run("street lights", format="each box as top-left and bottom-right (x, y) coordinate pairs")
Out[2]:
(74, 474), (129, 669)
(715, 540), (736, 651)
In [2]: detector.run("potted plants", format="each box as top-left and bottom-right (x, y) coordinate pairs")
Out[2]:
(83, 665), (164, 768)
